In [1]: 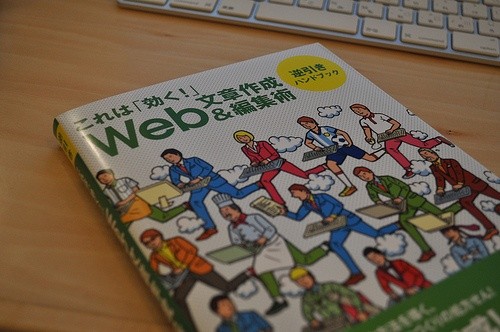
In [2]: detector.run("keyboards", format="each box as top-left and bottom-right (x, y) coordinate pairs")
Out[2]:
(117, 0), (500, 66)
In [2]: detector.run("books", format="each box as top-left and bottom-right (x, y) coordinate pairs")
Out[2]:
(52, 41), (500, 331)
